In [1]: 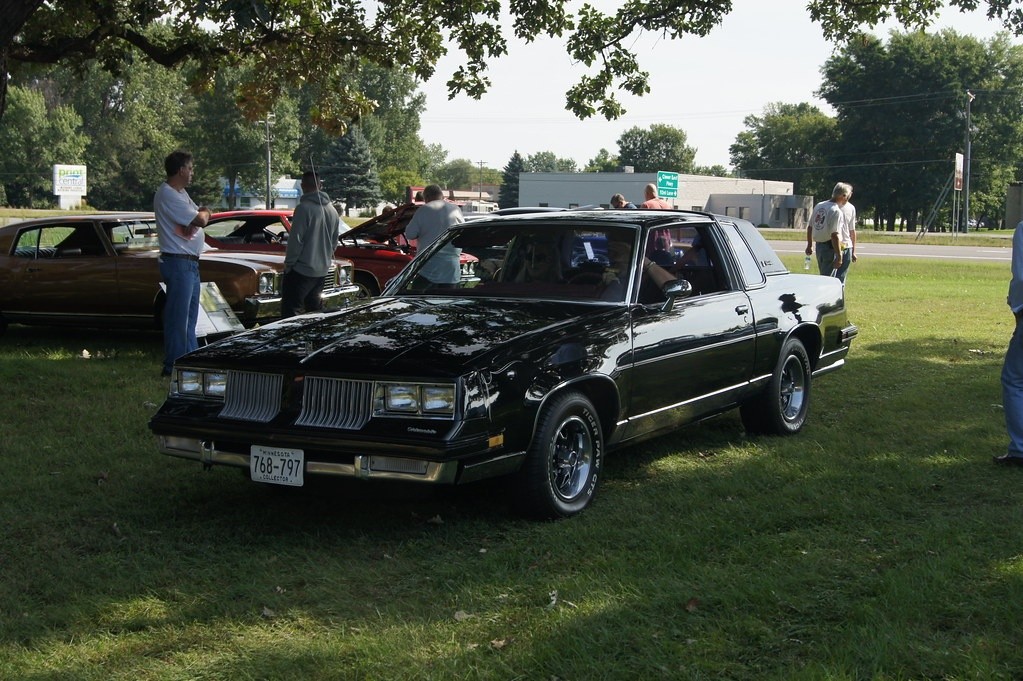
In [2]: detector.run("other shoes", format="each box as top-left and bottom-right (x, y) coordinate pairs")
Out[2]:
(160, 367), (172, 377)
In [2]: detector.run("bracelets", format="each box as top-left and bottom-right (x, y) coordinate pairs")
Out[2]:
(645, 261), (657, 271)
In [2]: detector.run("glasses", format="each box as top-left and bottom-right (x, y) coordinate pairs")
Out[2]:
(524, 253), (547, 262)
(615, 203), (620, 208)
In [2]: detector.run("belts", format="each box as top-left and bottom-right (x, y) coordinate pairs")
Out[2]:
(161, 252), (200, 262)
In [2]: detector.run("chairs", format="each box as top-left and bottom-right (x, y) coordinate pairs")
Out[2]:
(245, 232), (268, 245)
(105, 244), (129, 257)
(52, 247), (82, 260)
(275, 231), (289, 245)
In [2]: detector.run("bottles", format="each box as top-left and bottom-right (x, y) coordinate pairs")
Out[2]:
(804, 253), (810, 270)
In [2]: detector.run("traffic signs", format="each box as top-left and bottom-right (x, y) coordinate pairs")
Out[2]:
(657, 171), (678, 199)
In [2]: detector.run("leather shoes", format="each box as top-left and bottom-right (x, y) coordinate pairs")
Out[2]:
(993, 453), (1022, 467)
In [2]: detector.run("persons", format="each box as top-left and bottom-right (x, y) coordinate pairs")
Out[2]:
(805, 182), (858, 288)
(415, 191), (425, 203)
(334, 205), (343, 216)
(404, 184), (466, 290)
(283, 170), (340, 317)
(640, 183), (673, 251)
(648, 236), (672, 259)
(589, 227), (683, 298)
(154, 151), (212, 377)
(689, 236), (710, 266)
(990, 222), (1023, 464)
(382, 205), (393, 215)
(610, 193), (638, 209)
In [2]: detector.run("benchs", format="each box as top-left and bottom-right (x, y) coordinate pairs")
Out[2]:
(662, 265), (717, 296)
(14, 248), (57, 258)
(211, 236), (246, 244)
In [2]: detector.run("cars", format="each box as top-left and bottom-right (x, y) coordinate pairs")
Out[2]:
(204, 202), (480, 297)
(146, 203), (859, 518)
(0, 214), (360, 342)
